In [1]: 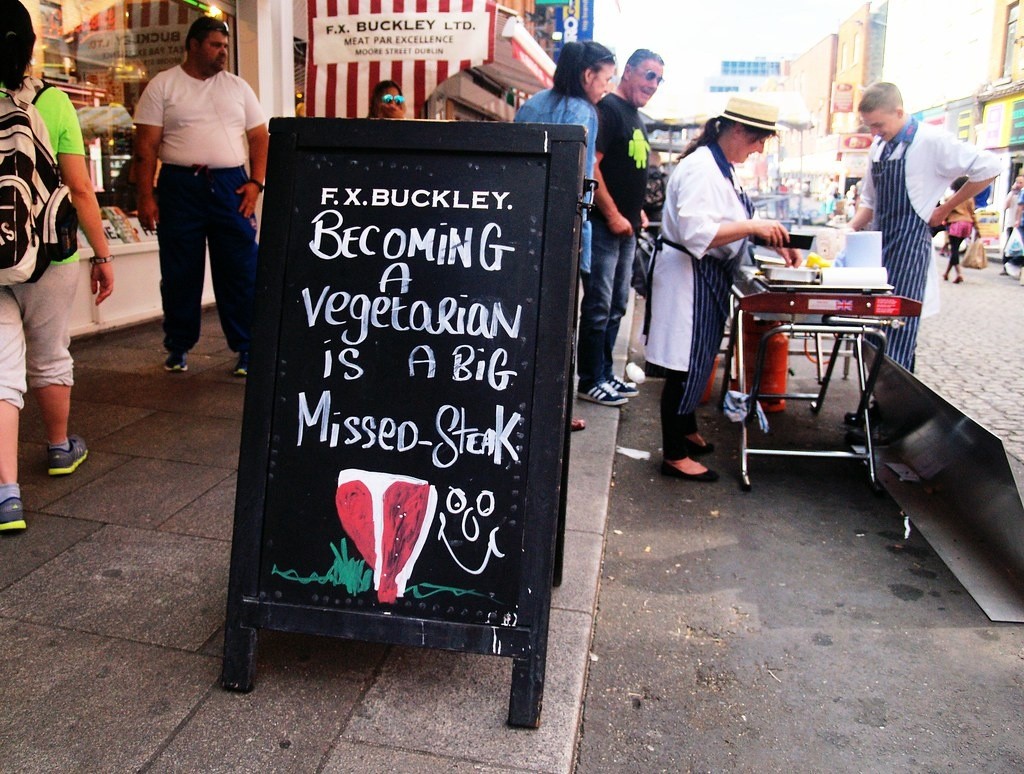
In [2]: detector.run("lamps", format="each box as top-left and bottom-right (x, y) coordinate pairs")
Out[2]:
(109, 27), (150, 84)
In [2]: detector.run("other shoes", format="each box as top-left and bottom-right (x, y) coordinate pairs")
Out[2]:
(943, 272), (949, 281)
(953, 276), (962, 283)
(844, 411), (864, 424)
(570, 418), (585, 431)
(846, 428), (871, 443)
(661, 459), (719, 481)
(683, 432), (714, 456)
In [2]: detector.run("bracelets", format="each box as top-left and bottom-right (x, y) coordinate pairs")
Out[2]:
(250, 179), (263, 193)
(90, 255), (114, 264)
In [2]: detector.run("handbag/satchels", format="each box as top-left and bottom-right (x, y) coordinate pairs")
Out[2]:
(961, 236), (988, 269)
(1004, 226), (1024, 257)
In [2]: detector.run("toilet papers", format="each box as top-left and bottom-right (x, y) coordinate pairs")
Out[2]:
(841, 230), (883, 267)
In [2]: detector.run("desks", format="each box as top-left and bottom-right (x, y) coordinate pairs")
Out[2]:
(720, 276), (903, 496)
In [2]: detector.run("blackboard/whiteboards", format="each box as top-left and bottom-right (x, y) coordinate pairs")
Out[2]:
(226, 116), (599, 658)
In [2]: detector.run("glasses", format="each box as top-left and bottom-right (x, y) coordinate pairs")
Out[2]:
(630, 64), (664, 85)
(378, 94), (405, 105)
(583, 53), (616, 72)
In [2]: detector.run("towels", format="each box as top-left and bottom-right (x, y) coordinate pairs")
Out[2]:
(723, 389), (770, 435)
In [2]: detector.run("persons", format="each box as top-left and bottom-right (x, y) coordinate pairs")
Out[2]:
(824, 176), (856, 219)
(577, 49), (665, 406)
(638, 97), (803, 481)
(514, 42), (615, 432)
(1005, 175), (1024, 286)
(843, 82), (1002, 442)
(369, 80), (406, 119)
(134, 17), (269, 375)
(944, 176), (981, 284)
(0, 0), (114, 530)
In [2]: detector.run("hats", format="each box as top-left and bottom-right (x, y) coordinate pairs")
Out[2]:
(720, 97), (779, 130)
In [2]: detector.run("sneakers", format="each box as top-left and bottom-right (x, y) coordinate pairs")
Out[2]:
(0, 500), (26, 530)
(234, 350), (248, 376)
(576, 379), (629, 406)
(164, 350), (187, 371)
(48, 434), (87, 474)
(605, 374), (640, 398)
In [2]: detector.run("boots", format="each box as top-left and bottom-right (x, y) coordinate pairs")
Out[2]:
(1019, 268), (1024, 286)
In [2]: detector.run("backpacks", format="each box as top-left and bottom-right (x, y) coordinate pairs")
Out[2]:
(0, 76), (78, 285)
